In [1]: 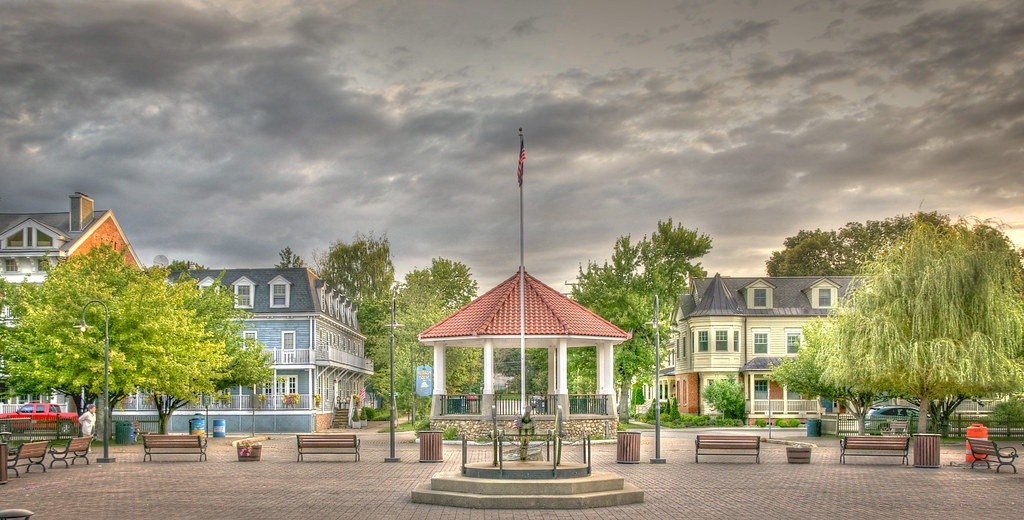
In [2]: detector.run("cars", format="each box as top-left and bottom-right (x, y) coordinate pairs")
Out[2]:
(467, 392), (478, 401)
(530, 396), (544, 408)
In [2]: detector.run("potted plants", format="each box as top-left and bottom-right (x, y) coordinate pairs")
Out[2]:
(351, 407), (368, 428)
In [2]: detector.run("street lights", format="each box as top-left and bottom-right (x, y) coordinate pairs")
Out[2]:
(644, 294), (667, 464)
(384, 298), (405, 463)
(73, 300), (109, 459)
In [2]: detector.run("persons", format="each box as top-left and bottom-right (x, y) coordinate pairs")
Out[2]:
(78, 405), (97, 454)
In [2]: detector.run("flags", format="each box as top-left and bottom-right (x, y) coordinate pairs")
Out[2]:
(517, 138), (526, 187)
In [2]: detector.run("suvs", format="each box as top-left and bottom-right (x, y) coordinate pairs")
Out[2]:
(864, 406), (952, 437)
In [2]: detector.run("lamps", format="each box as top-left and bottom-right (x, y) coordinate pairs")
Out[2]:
(0, 432), (12, 442)
(0, 508), (35, 520)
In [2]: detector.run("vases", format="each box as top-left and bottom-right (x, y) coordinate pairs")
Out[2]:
(237, 443), (262, 461)
(786, 447), (811, 463)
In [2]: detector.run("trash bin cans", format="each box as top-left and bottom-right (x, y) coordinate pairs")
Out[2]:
(189, 417), (206, 437)
(913, 433), (942, 468)
(116, 420), (131, 444)
(0, 443), (8, 485)
(616, 431), (642, 464)
(807, 419), (821, 437)
(419, 430), (445, 462)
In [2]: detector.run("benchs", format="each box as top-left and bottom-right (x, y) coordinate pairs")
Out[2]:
(695, 435), (760, 464)
(966, 437), (1019, 474)
(882, 420), (909, 436)
(48, 436), (93, 468)
(297, 434), (360, 462)
(840, 435), (911, 466)
(7, 442), (49, 478)
(141, 434), (207, 462)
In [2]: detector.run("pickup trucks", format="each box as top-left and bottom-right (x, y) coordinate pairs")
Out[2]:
(0, 404), (80, 435)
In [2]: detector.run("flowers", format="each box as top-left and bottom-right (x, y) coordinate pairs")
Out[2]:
(281, 393), (300, 405)
(240, 441), (254, 457)
